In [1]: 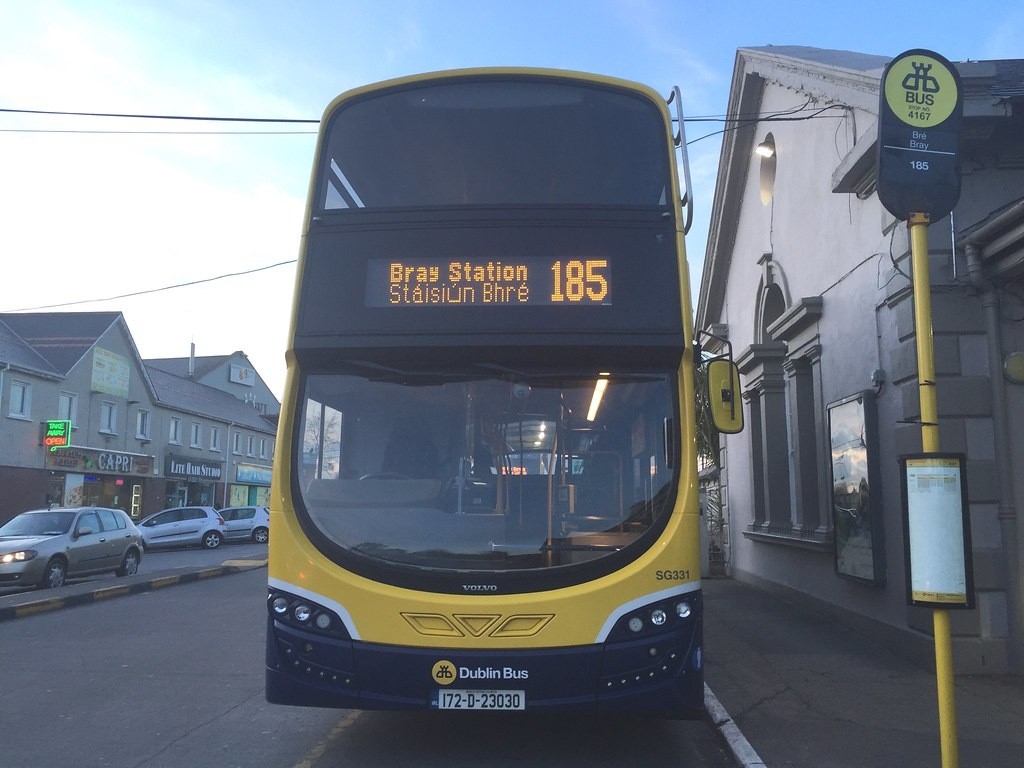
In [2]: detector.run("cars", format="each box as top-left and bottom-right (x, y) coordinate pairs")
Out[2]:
(217, 506), (270, 544)
(0, 504), (145, 589)
(136, 505), (227, 549)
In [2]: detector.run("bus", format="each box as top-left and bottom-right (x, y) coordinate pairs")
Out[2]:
(260, 64), (747, 717)
